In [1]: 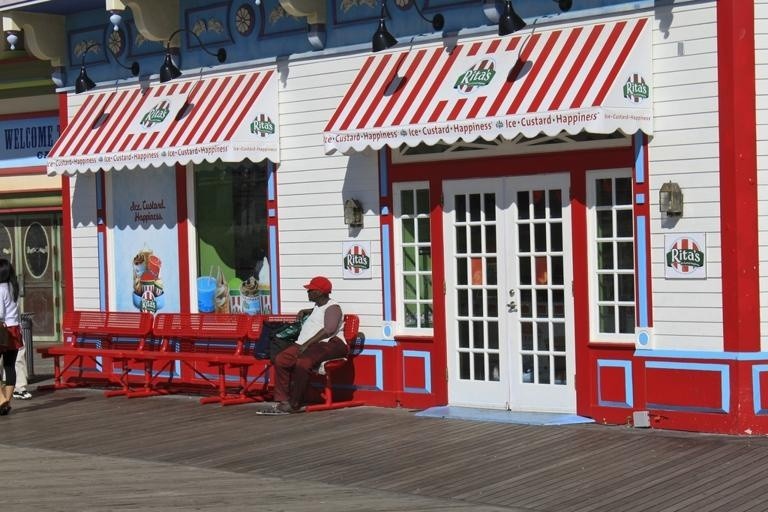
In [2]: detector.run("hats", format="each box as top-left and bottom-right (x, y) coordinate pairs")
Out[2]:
(303, 277), (332, 294)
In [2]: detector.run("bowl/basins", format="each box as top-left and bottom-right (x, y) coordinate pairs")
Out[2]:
(132, 291), (166, 310)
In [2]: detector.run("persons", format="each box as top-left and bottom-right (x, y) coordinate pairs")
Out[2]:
(0, 258), (23, 416)
(272, 277), (346, 414)
(12, 338), (32, 401)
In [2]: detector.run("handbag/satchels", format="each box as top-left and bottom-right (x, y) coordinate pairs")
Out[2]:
(253, 320), (287, 359)
(271, 315), (312, 340)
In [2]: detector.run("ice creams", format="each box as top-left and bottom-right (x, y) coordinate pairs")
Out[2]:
(214, 256), (273, 317)
(132, 244), (163, 301)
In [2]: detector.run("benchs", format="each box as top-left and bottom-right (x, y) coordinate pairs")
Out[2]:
(305, 313), (366, 412)
(36, 312), (298, 407)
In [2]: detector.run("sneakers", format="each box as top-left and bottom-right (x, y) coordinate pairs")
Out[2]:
(256, 405), (291, 415)
(12, 390), (31, 400)
(1, 404), (10, 414)
(286, 406), (305, 413)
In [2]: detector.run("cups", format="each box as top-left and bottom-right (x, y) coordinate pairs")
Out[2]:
(140, 251), (153, 267)
(240, 280), (262, 316)
(140, 280), (164, 296)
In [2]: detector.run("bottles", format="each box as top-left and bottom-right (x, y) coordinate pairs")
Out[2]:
(215, 296), (231, 314)
(197, 275), (216, 315)
(134, 255), (145, 293)
(148, 255), (161, 278)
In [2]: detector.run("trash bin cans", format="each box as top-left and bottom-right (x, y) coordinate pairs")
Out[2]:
(20, 312), (36, 384)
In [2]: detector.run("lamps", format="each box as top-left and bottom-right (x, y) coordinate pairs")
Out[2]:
(103, 1), (127, 33)
(497, 1), (575, 38)
(343, 197), (362, 226)
(158, 28), (228, 84)
(1, 14), (23, 50)
(658, 180), (683, 214)
(370, 0), (447, 54)
(73, 41), (142, 94)
